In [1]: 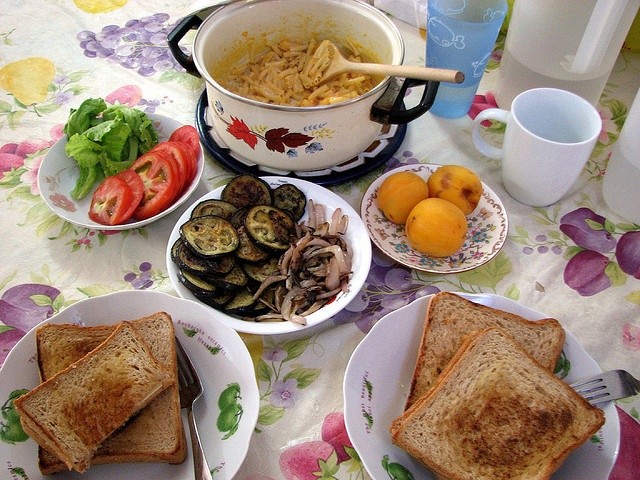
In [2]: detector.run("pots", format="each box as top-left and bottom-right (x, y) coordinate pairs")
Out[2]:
(166, 0), (441, 171)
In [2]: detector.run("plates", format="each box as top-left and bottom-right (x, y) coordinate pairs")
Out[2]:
(341, 290), (622, 480)
(36, 113), (205, 232)
(0, 288), (261, 480)
(164, 174), (374, 336)
(360, 162), (510, 275)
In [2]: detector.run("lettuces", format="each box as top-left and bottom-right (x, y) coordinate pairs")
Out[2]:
(62, 96), (157, 199)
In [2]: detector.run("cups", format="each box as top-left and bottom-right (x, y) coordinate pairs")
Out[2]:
(424, 0), (508, 119)
(602, 87), (640, 226)
(470, 87), (604, 208)
(492, 0), (640, 113)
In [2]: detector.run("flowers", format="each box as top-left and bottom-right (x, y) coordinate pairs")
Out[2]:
(34, 69), (89, 110)
(584, 86), (629, 178)
(0, 148), (149, 252)
(621, 291), (640, 352)
(255, 334), (322, 435)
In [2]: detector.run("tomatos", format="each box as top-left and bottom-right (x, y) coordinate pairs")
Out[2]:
(89, 125), (200, 227)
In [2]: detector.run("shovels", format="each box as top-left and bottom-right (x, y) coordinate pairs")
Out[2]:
(318, 42), (465, 84)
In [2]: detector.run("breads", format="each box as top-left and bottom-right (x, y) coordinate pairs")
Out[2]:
(13, 322), (177, 474)
(36, 313), (188, 476)
(405, 291), (566, 411)
(391, 326), (605, 476)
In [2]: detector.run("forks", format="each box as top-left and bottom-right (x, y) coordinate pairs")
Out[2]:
(568, 369), (640, 406)
(172, 335), (213, 480)
